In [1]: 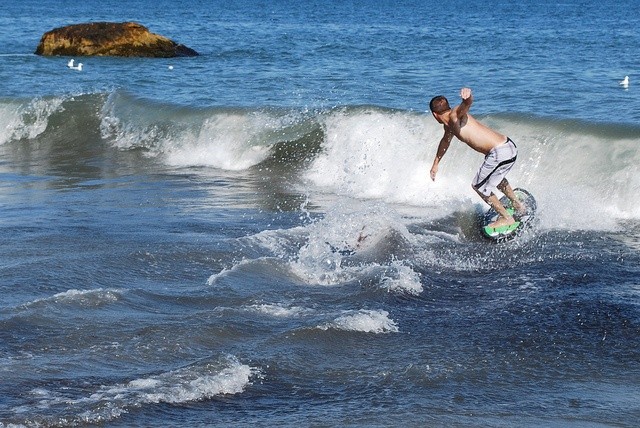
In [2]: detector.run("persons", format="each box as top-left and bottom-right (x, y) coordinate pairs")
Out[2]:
(430, 88), (526, 228)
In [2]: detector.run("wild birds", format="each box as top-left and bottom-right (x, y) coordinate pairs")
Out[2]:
(67, 58), (74, 68)
(619, 76), (630, 88)
(70, 62), (83, 71)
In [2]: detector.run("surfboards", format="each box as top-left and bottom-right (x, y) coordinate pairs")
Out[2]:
(479, 188), (537, 244)
